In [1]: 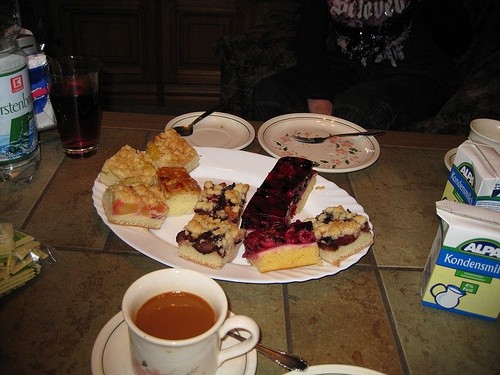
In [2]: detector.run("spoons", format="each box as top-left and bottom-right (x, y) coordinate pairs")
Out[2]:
(172, 106), (217, 136)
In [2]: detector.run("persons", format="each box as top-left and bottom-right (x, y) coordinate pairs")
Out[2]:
(247, 0), (467, 137)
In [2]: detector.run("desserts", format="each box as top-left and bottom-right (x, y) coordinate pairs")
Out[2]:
(96, 127), (376, 273)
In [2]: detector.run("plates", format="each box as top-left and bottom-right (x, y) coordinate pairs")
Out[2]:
(279, 363), (387, 375)
(90, 308), (259, 375)
(444, 147), (458, 171)
(257, 112), (380, 174)
(164, 111), (256, 150)
(91, 146), (373, 285)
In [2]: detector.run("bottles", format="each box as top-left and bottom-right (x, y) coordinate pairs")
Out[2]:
(0, 35), (42, 188)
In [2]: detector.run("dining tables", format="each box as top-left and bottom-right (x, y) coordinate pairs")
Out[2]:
(0, 109), (500, 375)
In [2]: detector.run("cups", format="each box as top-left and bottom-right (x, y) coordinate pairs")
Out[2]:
(42, 54), (104, 160)
(469, 118), (500, 155)
(122, 267), (261, 375)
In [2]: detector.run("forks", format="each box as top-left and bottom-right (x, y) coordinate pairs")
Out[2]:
(291, 130), (387, 144)
